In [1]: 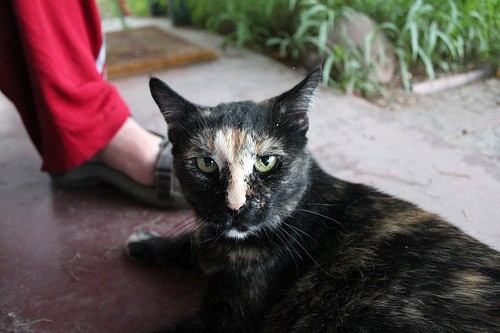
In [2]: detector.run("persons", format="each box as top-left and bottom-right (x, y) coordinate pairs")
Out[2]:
(0, 0), (191, 209)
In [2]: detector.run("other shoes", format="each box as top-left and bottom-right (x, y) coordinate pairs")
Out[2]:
(45, 128), (195, 211)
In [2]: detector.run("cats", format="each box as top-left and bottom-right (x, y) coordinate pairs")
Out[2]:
(122, 65), (500, 333)
